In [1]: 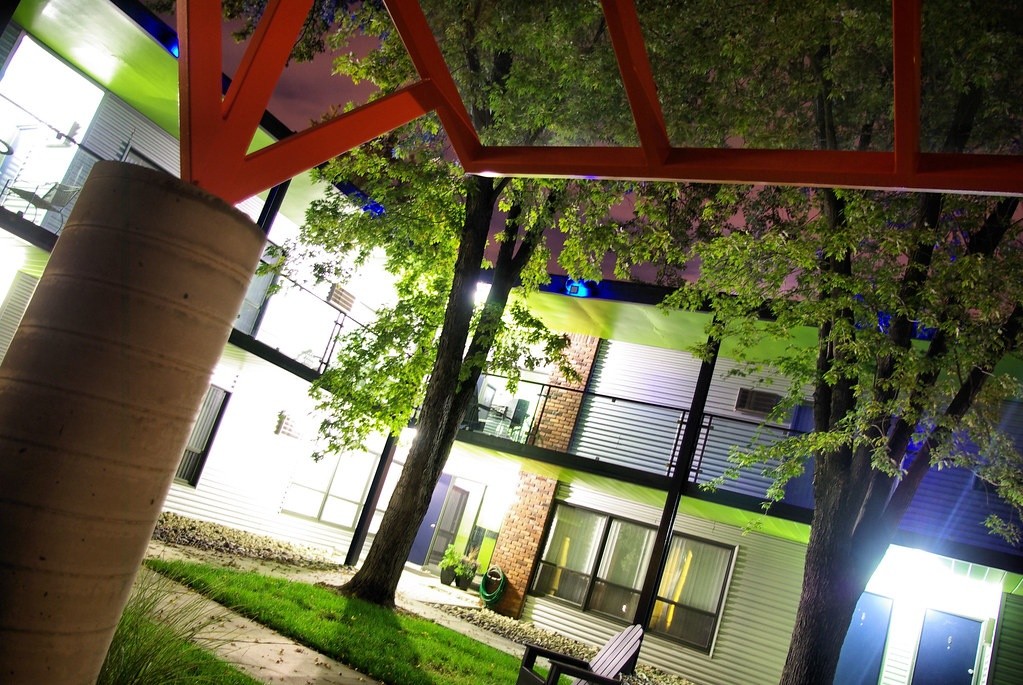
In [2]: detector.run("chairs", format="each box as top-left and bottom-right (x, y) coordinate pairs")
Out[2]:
(515, 623), (644, 685)
(0, 178), (81, 235)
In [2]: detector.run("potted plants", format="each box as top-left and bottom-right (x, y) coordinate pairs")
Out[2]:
(440, 542), (480, 591)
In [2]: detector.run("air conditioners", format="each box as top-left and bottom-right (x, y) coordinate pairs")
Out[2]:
(735, 385), (781, 417)
